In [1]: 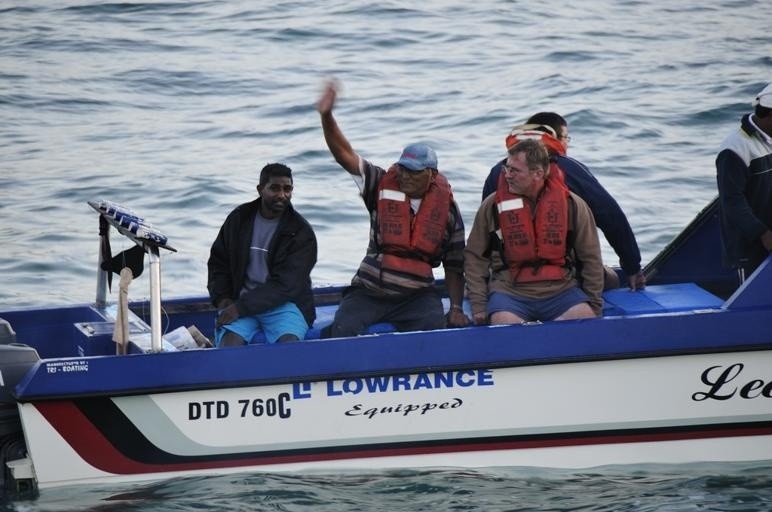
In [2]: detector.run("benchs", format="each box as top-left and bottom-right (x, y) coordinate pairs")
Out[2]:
(252, 280), (726, 342)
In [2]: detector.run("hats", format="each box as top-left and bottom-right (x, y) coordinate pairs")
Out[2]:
(756, 83), (772, 108)
(396, 143), (438, 172)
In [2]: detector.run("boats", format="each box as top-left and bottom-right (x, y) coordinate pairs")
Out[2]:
(0, 191), (771, 503)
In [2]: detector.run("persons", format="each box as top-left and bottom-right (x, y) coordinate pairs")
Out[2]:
(462, 140), (605, 327)
(715, 84), (771, 291)
(481, 111), (646, 293)
(206, 164), (318, 349)
(314, 79), (467, 339)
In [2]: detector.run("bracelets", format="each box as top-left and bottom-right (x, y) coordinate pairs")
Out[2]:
(450, 304), (463, 312)
